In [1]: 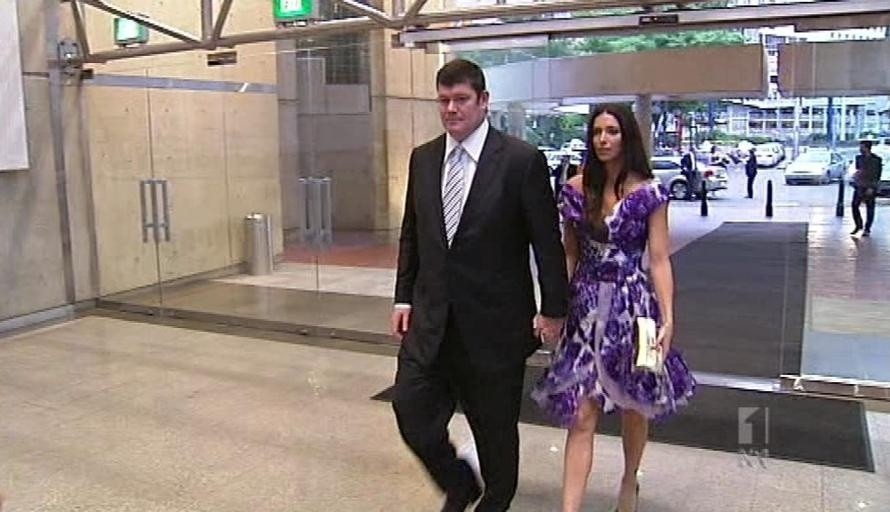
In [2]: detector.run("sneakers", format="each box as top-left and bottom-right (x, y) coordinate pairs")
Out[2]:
(851, 225), (869, 237)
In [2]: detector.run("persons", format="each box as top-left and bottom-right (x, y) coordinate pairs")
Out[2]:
(848, 138), (884, 237)
(549, 154), (579, 223)
(389, 54), (572, 510)
(532, 99), (698, 511)
(680, 145), (698, 199)
(746, 148), (758, 200)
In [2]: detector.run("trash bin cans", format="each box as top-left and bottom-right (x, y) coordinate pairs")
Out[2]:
(243, 211), (274, 277)
(296, 175), (336, 248)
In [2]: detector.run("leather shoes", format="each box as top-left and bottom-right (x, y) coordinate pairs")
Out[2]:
(440, 478), (513, 511)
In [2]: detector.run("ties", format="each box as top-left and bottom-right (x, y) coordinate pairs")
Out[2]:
(442, 145), (464, 245)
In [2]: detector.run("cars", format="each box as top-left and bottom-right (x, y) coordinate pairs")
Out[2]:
(784, 149), (847, 185)
(535, 137), (589, 182)
(642, 142), (784, 198)
